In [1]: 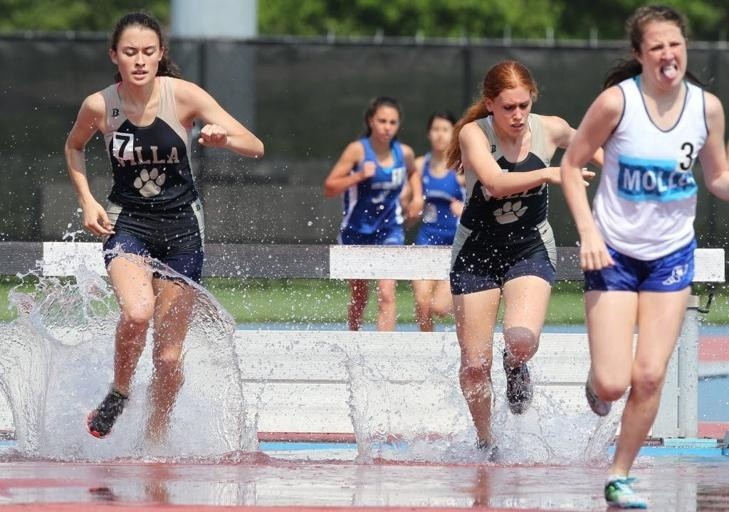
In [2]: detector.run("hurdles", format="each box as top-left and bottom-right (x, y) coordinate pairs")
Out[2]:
(0, 241), (728, 448)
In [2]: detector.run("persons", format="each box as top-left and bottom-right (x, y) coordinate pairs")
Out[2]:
(559, 4), (729, 510)
(447, 61), (614, 463)
(320, 95), (416, 332)
(64, 15), (265, 443)
(404, 113), (465, 331)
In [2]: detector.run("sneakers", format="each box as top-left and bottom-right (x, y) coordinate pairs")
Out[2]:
(583, 362), (613, 417)
(472, 437), (500, 464)
(85, 386), (131, 440)
(501, 347), (536, 415)
(605, 475), (648, 509)
